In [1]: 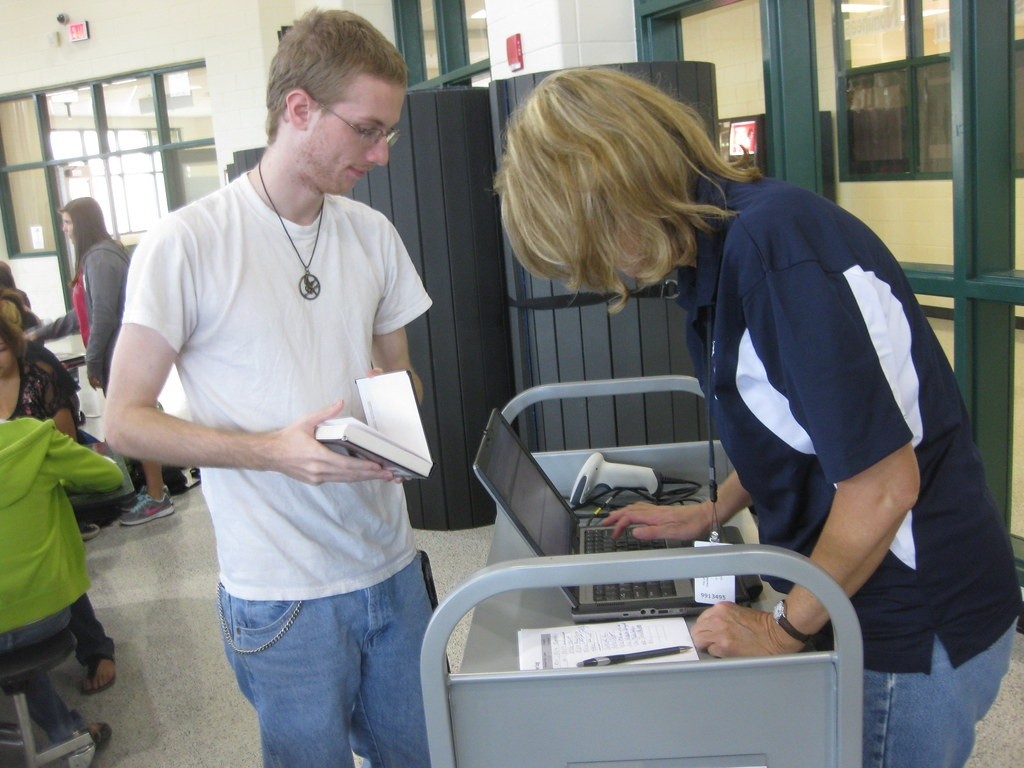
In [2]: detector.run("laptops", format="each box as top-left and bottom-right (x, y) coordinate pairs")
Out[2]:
(472, 407), (751, 624)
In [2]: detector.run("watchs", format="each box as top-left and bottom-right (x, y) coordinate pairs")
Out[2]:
(771, 600), (812, 645)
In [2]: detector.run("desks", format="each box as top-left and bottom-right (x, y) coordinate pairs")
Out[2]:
(417, 373), (866, 768)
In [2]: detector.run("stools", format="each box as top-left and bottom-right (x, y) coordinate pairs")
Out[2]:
(0, 626), (99, 768)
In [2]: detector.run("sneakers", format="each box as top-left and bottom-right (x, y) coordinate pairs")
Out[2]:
(119, 493), (175, 525)
(79, 523), (101, 540)
(120, 484), (174, 512)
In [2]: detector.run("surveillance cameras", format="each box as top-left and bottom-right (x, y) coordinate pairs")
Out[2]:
(56, 13), (70, 25)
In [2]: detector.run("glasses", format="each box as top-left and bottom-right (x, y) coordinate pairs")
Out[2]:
(313, 96), (400, 148)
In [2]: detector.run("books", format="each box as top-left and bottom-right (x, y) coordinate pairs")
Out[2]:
(314, 368), (433, 479)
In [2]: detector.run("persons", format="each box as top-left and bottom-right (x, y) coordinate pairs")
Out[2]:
(493, 69), (1022, 768)
(104, 7), (434, 768)
(0, 260), (125, 752)
(22, 197), (175, 526)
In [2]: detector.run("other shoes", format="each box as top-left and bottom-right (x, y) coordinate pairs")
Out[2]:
(86, 722), (113, 745)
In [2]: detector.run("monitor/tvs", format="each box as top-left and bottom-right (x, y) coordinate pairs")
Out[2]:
(728, 120), (760, 156)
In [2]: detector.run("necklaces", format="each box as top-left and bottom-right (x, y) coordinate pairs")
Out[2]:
(258, 158), (323, 299)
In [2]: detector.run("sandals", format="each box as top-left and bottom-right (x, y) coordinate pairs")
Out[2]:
(81, 653), (117, 695)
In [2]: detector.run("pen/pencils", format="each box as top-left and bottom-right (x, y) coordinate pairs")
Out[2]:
(578, 646), (691, 666)
